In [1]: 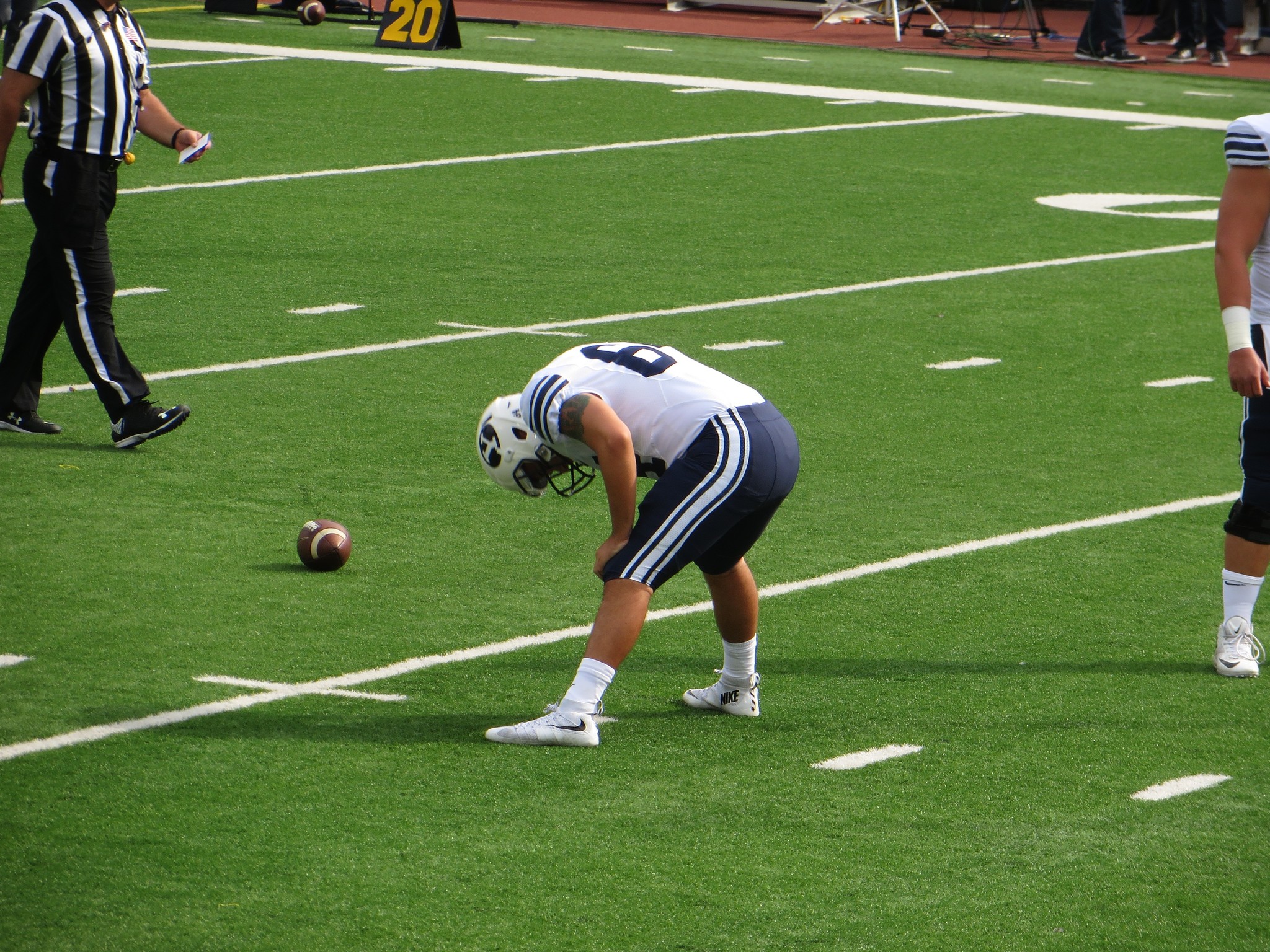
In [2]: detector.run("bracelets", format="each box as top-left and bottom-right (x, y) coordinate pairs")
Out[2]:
(171, 128), (186, 150)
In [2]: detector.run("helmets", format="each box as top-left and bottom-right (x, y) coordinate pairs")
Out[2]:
(476, 393), (557, 496)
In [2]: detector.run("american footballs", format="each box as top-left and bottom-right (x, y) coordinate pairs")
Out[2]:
(296, 0), (325, 26)
(297, 519), (352, 572)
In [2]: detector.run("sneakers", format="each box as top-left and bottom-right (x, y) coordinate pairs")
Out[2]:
(1213, 615), (1260, 678)
(1073, 28), (1230, 67)
(484, 697), (600, 746)
(682, 670), (760, 716)
(110, 400), (190, 448)
(0, 410), (61, 434)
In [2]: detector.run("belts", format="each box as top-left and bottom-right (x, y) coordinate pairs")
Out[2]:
(33, 139), (124, 173)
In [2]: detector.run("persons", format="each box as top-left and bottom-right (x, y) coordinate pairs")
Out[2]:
(1213, 110), (1269, 680)
(0, 0), (212, 449)
(1070, 0), (1270, 66)
(475, 340), (801, 750)
